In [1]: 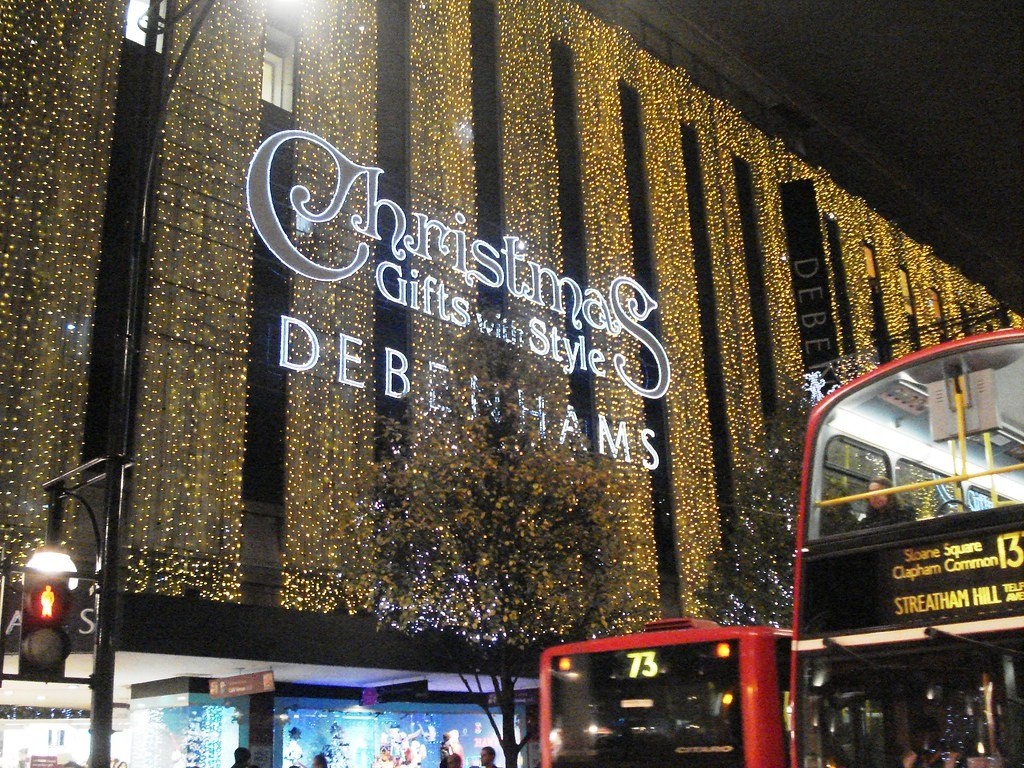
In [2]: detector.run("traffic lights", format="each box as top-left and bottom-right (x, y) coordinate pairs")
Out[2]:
(16, 572), (71, 680)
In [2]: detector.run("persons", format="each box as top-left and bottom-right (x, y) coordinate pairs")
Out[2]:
(439, 733), (461, 768)
(820, 486), (858, 536)
(898, 717), (965, 768)
(481, 747), (498, 768)
(314, 755), (327, 768)
(854, 477), (915, 531)
(232, 747), (259, 768)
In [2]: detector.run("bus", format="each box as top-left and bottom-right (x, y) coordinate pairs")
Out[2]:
(784, 324), (1024, 768)
(535, 614), (883, 768)
